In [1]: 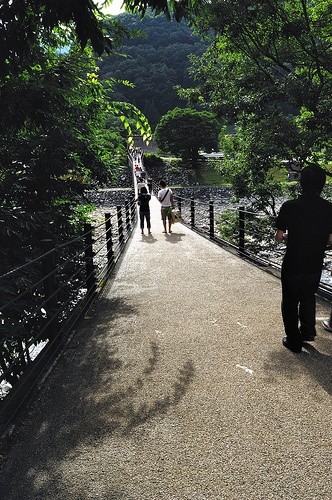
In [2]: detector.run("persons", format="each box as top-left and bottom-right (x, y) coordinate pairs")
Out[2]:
(158, 179), (173, 233)
(132, 146), (154, 205)
(135, 186), (152, 235)
(322, 310), (332, 332)
(273, 164), (332, 353)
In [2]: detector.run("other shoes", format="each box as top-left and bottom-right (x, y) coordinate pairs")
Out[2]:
(141, 233), (144, 234)
(148, 232), (152, 235)
(168, 230), (172, 233)
(162, 231), (167, 233)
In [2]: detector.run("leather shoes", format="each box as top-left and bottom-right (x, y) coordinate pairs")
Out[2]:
(299, 331), (315, 341)
(282, 336), (302, 354)
